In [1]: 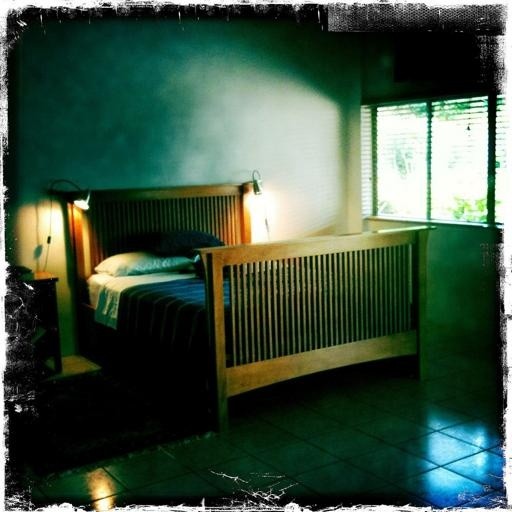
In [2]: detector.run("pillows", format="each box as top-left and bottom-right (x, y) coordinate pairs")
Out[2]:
(93, 230), (225, 277)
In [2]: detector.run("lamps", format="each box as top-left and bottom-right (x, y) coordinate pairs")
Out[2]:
(252, 169), (261, 196)
(50, 179), (91, 211)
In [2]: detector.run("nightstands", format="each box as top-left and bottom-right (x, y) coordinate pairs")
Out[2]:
(26, 269), (63, 373)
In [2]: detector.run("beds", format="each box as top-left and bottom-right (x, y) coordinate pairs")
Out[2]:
(60, 183), (437, 432)
